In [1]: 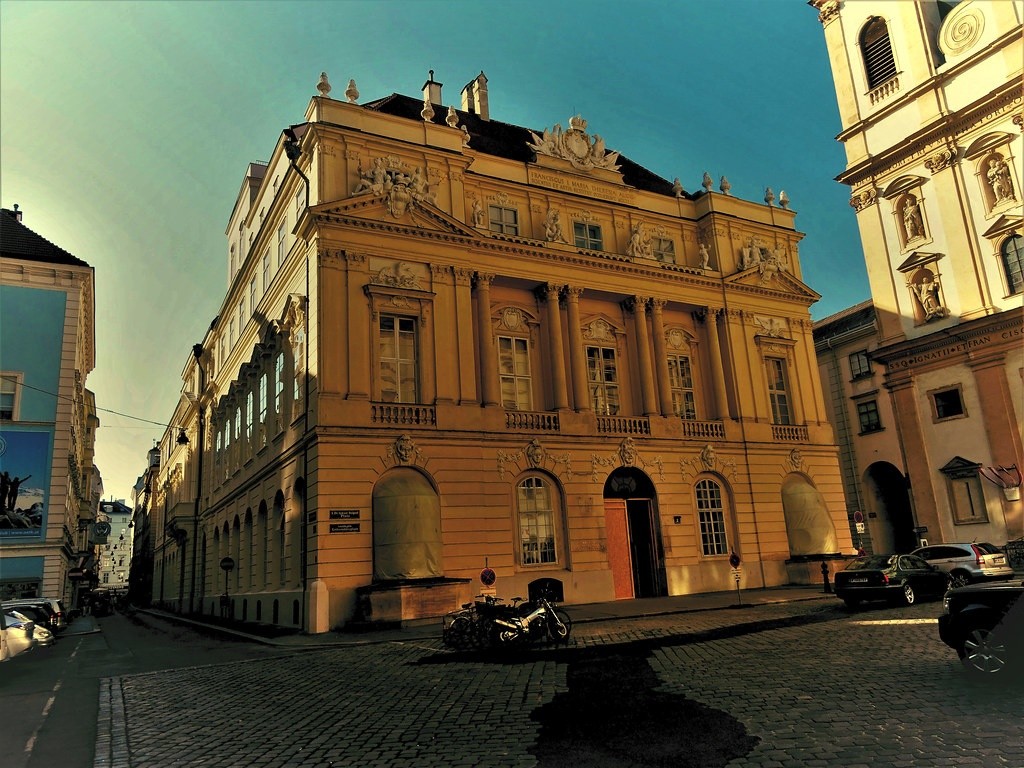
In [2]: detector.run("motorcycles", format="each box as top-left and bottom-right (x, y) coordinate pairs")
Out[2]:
(495, 582), (569, 647)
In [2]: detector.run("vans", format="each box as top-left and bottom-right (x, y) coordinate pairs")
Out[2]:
(0, 610), (8, 662)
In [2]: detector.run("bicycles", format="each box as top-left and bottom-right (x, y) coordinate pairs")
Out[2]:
(443, 590), (572, 648)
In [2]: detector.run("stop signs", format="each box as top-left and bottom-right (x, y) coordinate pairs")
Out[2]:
(69, 568), (83, 582)
(220, 557), (234, 571)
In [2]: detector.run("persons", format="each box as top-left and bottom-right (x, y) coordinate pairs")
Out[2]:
(542, 206), (571, 245)
(352, 155), (441, 218)
(469, 198), (488, 230)
(737, 235), (788, 285)
(625, 225), (658, 260)
(9, 474), (33, 514)
(0, 471), (11, 515)
(697, 242), (714, 270)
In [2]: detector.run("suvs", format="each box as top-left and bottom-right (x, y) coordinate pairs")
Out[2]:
(909, 542), (1015, 589)
(1, 598), (67, 660)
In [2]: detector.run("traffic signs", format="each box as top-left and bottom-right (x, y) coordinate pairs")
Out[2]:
(913, 526), (928, 533)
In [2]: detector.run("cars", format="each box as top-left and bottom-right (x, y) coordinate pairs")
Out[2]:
(835, 553), (955, 609)
(938, 579), (1024, 679)
(4, 614), (34, 658)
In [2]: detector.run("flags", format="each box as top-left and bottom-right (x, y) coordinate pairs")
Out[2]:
(978, 467), (1018, 489)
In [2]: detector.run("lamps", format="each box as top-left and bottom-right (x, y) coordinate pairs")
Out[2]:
(281, 128), (309, 206)
(119, 534), (125, 542)
(128, 519), (134, 527)
(114, 544), (117, 549)
(176, 427), (189, 446)
(144, 483), (151, 494)
